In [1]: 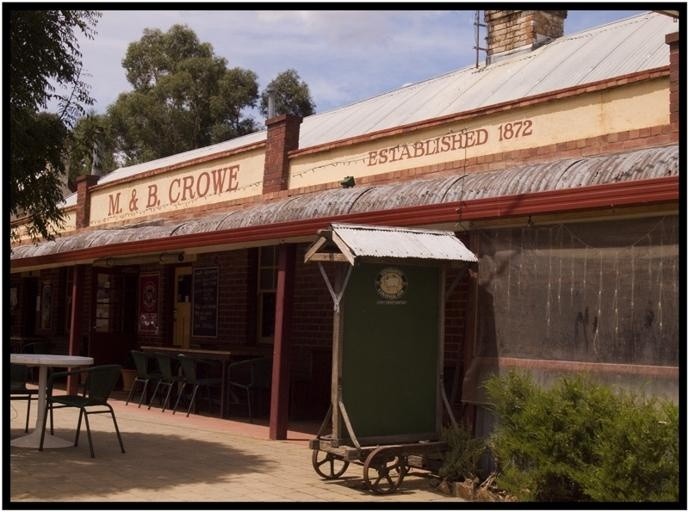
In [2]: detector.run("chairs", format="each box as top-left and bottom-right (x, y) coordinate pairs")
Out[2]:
(2, 330), (128, 458)
(124, 344), (268, 424)
